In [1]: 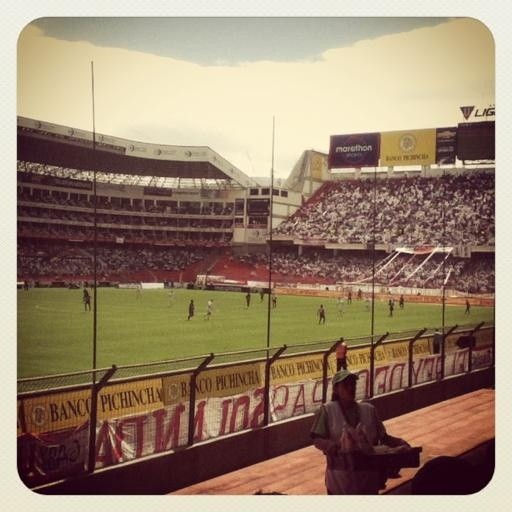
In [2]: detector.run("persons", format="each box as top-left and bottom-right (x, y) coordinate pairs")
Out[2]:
(317, 304), (325, 325)
(310, 370), (411, 495)
(260, 288), (264, 301)
(246, 292), (251, 307)
(357, 288), (361, 300)
(272, 293), (277, 308)
(23, 279), (29, 296)
(82, 290), (92, 312)
(465, 300), (471, 315)
(388, 300), (394, 315)
(205, 298), (215, 321)
(348, 291), (352, 304)
(336, 336), (347, 373)
(188, 300), (194, 320)
(400, 294), (404, 308)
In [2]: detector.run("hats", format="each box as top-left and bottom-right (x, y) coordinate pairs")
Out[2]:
(332, 370), (359, 386)
